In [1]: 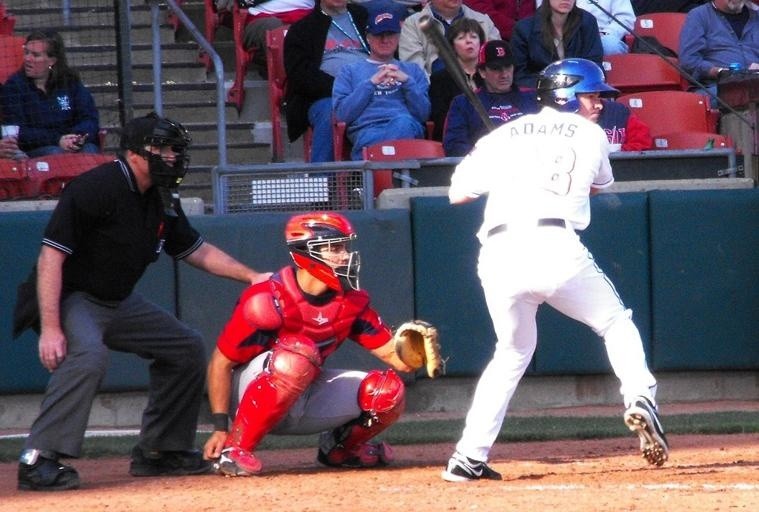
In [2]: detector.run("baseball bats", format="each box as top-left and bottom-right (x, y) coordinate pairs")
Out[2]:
(419, 15), (495, 130)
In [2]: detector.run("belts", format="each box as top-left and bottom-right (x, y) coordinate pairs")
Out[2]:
(488, 218), (565, 236)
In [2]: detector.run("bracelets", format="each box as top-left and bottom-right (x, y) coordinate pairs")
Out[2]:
(212, 413), (228, 431)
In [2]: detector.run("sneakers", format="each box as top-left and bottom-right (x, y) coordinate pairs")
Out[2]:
(318, 442), (389, 468)
(130, 444), (211, 476)
(624, 396), (669, 466)
(442, 451), (501, 481)
(213, 446), (252, 477)
(17, 450), (80, 491)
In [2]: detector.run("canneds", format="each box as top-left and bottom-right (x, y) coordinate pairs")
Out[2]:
(729, 62), (742, 75)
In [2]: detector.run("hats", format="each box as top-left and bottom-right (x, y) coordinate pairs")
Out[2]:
(365, 7), (400, 36)
(478, 40), (514, 66)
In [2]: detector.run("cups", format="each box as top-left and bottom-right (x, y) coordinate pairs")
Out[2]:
(0, 125), (20, 142)
(608, 143), (623, 152)
(728, 63), (742, 71)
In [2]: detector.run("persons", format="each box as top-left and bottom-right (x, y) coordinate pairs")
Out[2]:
(0, 135), (18, 160)
(202, 211), (446, 477)
(12, 111), (274, 491)
(439, 58), (668, 482)
(0, 30), (101, 159)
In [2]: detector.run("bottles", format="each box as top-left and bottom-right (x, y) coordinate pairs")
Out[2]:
(704, 138), (716, 150)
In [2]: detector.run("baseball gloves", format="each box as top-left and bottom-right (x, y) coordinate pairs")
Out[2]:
(395, 320), (443, 379)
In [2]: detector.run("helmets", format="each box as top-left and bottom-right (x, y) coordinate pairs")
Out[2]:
(536, 58), (622, 114)
(120, 112), (192, 188)
(285, 211), (361, 293)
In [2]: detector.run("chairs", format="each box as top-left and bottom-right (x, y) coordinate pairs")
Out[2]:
(602, 13), (736, 151)
(0, 0), (115, 200)
(171, 0), (446, 206)
